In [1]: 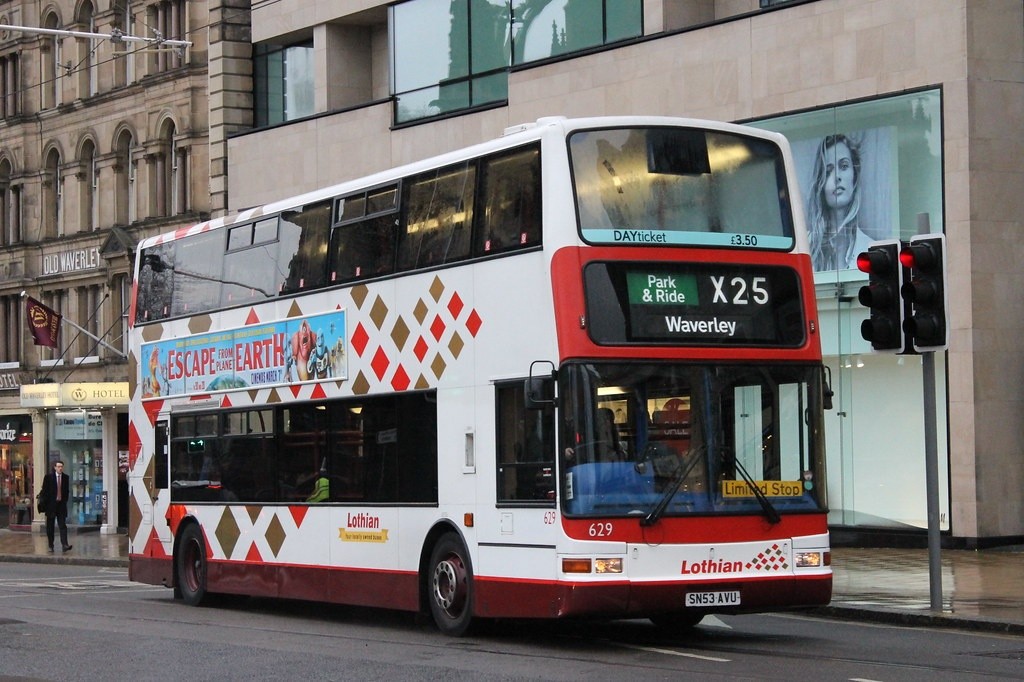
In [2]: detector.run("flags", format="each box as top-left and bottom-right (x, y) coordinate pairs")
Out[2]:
(26, 298), (58, 347)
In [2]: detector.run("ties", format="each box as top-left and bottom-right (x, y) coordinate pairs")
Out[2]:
(57, 476), (61, 500)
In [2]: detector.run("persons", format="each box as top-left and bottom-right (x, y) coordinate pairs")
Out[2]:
(44, 461), (72, 553)
(804, 135), (881, 272)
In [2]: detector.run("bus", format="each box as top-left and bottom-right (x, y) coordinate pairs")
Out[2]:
(124, 115), (831, 638)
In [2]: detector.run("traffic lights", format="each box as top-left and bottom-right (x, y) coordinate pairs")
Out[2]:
(856, 240), (905, 355)
(901, 234), (953, 354)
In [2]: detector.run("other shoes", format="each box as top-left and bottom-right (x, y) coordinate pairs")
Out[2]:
(49, 547), (54, 552)
(62, 545), (72, 552)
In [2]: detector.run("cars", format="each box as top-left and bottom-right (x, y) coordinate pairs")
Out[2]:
(172, 479), (223, 502)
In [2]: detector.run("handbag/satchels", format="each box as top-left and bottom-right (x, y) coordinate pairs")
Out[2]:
(37, 495), (56, 513)
